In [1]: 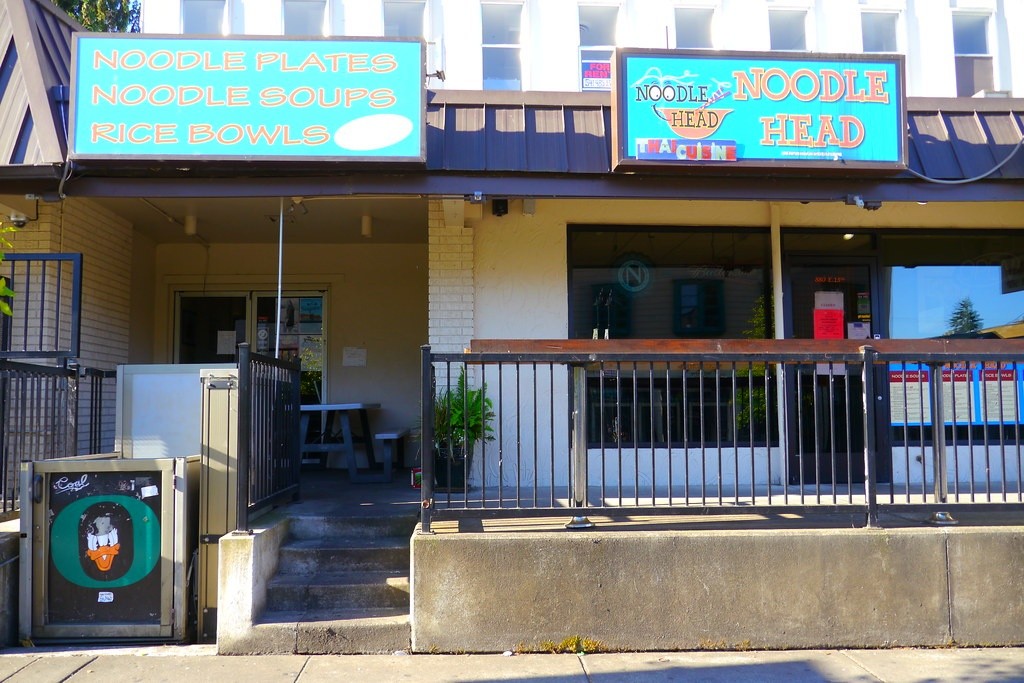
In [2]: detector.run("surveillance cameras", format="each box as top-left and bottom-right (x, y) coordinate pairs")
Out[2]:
(10, 214), (29, 228)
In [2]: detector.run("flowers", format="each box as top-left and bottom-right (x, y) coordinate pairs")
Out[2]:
(407, 347), (498, 465)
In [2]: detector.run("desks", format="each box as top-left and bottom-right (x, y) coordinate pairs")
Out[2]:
(290, 399), (382, 479)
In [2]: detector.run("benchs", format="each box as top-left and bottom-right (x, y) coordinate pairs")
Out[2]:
(373, 425), (413, 478)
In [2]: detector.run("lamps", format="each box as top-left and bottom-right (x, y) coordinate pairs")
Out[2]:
(184, 215), (197, 236)
(842, 193), (863, 205)
(361, 216), (373, 239)
(864, 200), (882, 211)
(469, 191), (487, 205)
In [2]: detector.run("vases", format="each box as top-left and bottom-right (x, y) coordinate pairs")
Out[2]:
(434, 446), (472, 488)
